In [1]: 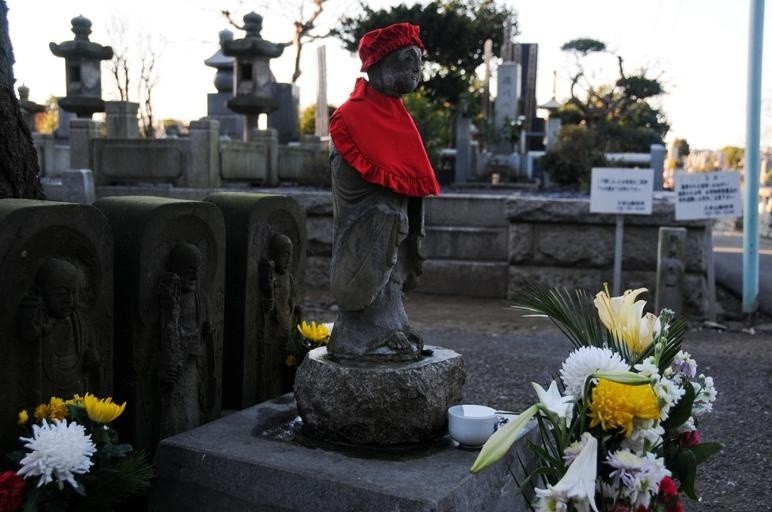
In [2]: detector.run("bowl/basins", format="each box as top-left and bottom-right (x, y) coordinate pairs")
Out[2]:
(448, 404), (498, 450)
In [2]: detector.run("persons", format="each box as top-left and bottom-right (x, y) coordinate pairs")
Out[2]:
(258, 232), (301, 400)
(19, 260), (103, 404)
(157, 243), (213, 439)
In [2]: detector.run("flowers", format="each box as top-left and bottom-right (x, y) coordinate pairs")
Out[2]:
(293, 320), (329, 350)
(0, 391), (128, 512)
(467, 282), (723, 511)
